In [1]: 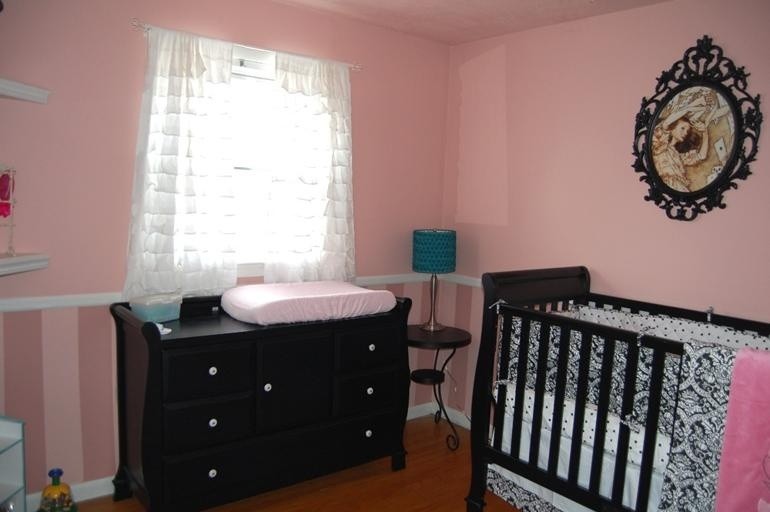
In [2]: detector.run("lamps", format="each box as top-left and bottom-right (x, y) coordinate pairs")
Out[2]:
(413, 228), (456, 332)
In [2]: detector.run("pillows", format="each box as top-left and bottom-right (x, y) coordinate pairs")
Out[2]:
(220, 280), (398, 328)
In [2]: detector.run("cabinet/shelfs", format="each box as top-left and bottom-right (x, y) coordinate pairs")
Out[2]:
(109, 295), (412, 512)
(0, 77), (51, 277)
(0, 416), (27, 512)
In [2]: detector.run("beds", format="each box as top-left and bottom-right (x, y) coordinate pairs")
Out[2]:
(463, 266), (770, 511)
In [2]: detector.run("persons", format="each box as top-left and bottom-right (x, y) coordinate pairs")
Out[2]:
(648, 96), (728, 196)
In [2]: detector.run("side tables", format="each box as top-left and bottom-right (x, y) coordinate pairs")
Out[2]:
(405, 324), (472, 451)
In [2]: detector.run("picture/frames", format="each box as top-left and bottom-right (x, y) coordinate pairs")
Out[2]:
(630, 35), (763, 222)
